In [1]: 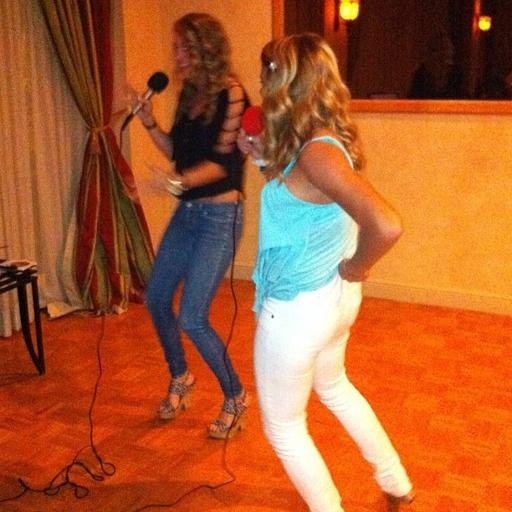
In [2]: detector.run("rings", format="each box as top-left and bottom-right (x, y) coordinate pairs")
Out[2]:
(247, 135), (253, 142)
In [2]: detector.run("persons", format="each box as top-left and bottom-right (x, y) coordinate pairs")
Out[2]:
(237, 31), (417, 511)
(122, 12), (253, 438)
(409, 34), (461, 98)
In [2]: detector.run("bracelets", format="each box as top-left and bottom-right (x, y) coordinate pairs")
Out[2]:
(142, 120), (157, 130)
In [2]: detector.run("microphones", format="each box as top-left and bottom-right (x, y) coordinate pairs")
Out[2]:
(240, 106), (267, 164)
(120, 72), (169, 131)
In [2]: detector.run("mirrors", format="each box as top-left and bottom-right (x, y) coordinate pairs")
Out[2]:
(272, 0), (512, 115)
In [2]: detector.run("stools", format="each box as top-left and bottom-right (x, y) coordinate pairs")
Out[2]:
(0, 259), (46, 375)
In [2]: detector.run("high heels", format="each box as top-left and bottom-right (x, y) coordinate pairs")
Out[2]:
(385, 489), (414, 511)
(158, 371), (198, 420)
(208, 390), (252, 439)
